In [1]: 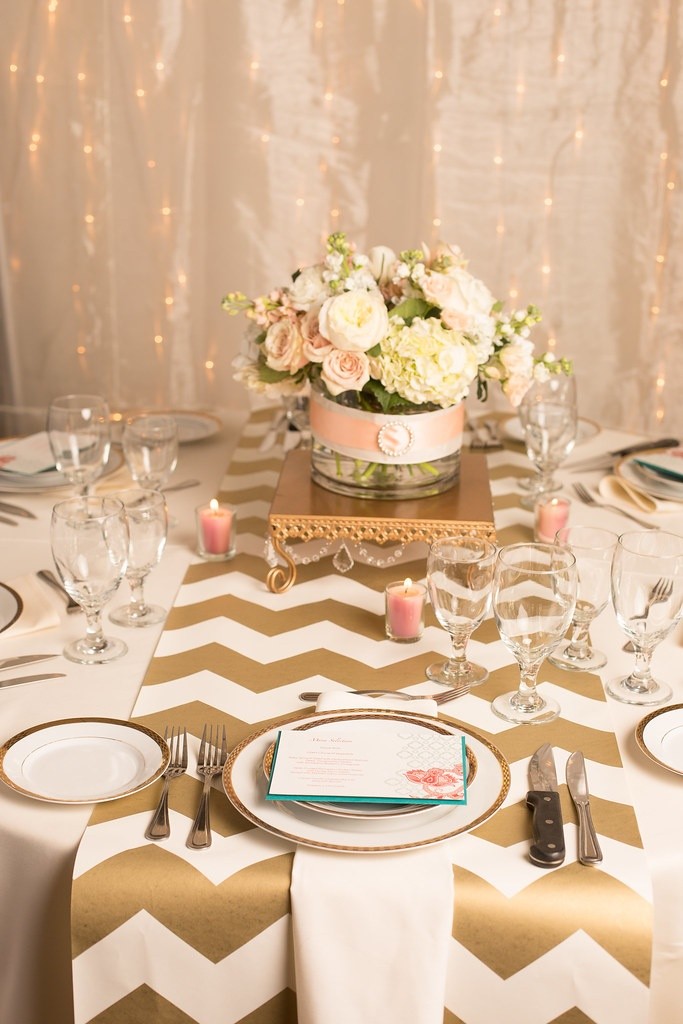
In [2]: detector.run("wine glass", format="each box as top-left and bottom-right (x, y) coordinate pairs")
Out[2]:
(38, 390), (183, 667)
(420, 370), (683, 723)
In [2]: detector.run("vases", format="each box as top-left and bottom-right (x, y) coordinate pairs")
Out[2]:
(311, 391), (463, 501)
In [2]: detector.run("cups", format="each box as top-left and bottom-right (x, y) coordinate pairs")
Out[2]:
(533, 491), (572, 552)
(385, 580), (428, 643)
(194, 501), (239, 562)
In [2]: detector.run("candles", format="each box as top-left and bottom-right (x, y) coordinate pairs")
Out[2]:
(199, 500), (232, 554)
(386, 578), (423, 638)
(538, 498), (570, 544)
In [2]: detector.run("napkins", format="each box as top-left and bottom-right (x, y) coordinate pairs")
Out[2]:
(289, 693), (454, 1024)
(0, 575), (60, 638)
(600, 475), (683, 514)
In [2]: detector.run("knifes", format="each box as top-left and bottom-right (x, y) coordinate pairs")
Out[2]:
(564, 751), (603, 866)
(556, 438), (678, 470)
(524, 742), (566, 869)
(1, 517), (18, 526)
(0, 501), (39, 519)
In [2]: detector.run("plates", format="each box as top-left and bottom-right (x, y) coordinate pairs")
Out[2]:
(622, 449), (683, 499)
(0, 717), (171, 805)
(501, 414), (600, 444)
(143, 409), (222, 444)
(0, 435), (124, 490)
(634, 703), (683, 778)
(219, 710), (511, 854)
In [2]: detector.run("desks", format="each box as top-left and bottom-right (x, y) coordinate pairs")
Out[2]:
(0, 408), (683, 1024)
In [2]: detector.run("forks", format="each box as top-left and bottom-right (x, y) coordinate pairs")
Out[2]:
(143, 726), (188, 840)
(295, 685), (468, 704)
(567, 480), (659, 531)
(184, 723), (228, 850)
(626, 581), (673, 652)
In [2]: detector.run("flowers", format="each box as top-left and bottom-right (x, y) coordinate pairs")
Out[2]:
(219, 236), (571, 481)
